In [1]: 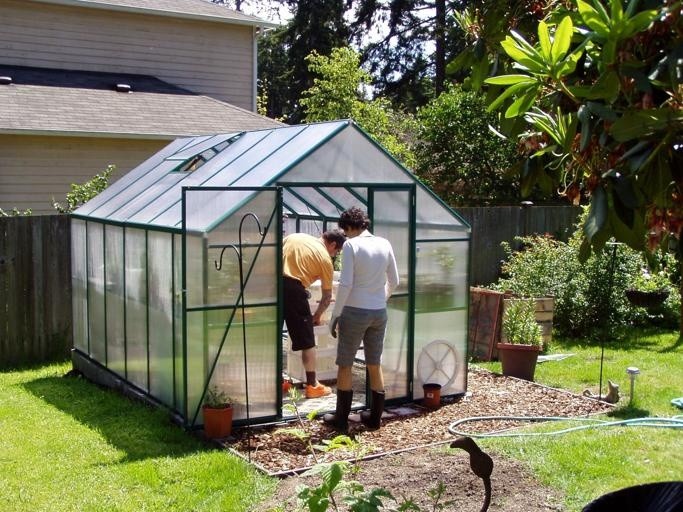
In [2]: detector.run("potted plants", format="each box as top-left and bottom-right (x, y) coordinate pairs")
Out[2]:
(422, 246), (457, 308)
(201, 385), (233, 439)
(489, 232), (570, 345)
(496, 297), (544, 381)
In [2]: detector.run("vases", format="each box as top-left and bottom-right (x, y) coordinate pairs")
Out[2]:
(625, 289), (670, 306)
(235, 310), (252, 323)
(423, 383), (441, 406)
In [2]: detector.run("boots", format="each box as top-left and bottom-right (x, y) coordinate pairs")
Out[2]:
(360, 389), (385, 430)
(323, 388), (352, 431)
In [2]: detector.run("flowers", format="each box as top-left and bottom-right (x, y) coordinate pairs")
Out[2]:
(631, 269), (670, 292)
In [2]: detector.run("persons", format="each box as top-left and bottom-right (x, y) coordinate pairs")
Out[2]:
(281, 227), (344, 399)
(323, 206), (400, 433)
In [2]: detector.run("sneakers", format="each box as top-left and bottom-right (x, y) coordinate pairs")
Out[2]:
(305, 380), (331, 398)
(282, 382), (292, 393)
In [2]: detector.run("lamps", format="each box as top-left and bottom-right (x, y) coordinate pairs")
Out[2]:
(117, 84), (131, 92)
(0, 76), (12, 85)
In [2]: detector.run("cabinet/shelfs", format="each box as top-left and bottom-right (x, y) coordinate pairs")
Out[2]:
(287, 280), (339, 387)
